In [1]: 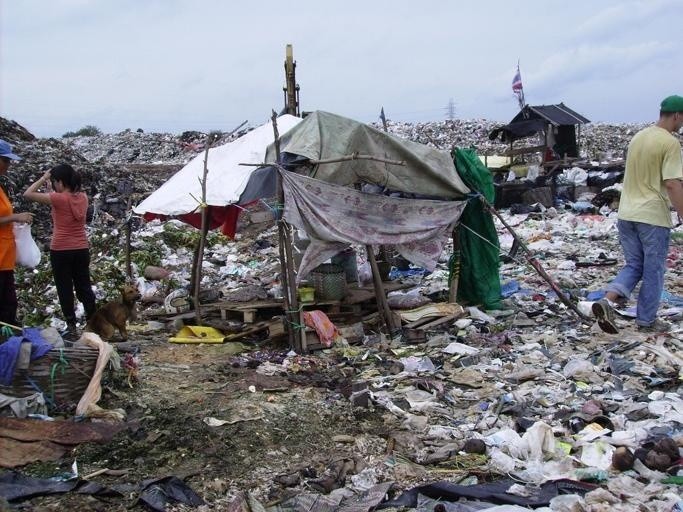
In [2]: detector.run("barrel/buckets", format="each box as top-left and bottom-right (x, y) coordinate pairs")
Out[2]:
(332, 249), (358, 282)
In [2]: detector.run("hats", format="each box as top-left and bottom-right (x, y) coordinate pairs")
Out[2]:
(659, 95), (682, 112)
(0, 139), (22, 161)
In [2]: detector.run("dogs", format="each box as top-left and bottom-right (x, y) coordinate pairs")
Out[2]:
(85, 282), (142, 342)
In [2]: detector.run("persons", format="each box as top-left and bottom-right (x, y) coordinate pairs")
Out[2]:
(591, 95), (682, 334)
(20, 161), (97, 344)
(0, 136), (37, 323)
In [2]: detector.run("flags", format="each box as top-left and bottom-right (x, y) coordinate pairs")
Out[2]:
(509, 57), (527, 115)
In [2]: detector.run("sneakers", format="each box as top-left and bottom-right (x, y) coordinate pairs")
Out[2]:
(637, 319), (670, 333)
(591, 299), (618, 334)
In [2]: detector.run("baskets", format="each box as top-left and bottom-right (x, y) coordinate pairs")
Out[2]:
(0, 347), (99, 411)
(307, 263), (347, 299)
